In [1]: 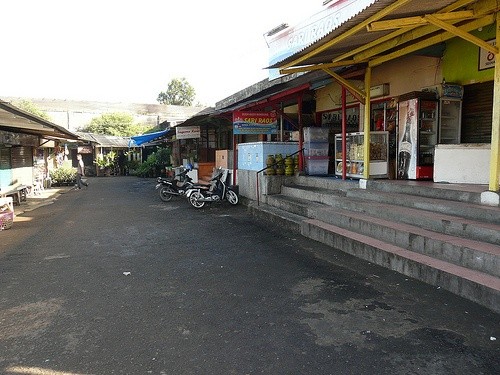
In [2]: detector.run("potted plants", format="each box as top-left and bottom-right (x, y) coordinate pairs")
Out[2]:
(92, 157), (114, 176)
(134, 146), (172, 178)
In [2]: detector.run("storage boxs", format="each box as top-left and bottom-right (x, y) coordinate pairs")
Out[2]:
(302, 126), (331, 175)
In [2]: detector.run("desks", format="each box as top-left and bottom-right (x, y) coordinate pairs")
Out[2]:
(433, 143), (491, 185)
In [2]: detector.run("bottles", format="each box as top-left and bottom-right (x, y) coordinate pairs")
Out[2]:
(396, 120), (412, 180)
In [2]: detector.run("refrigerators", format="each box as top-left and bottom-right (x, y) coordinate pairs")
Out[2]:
(437, 85), (464, 143)
(395, 91), (439, 180)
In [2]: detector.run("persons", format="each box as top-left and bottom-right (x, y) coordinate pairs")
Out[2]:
(75, 154), (89, 190)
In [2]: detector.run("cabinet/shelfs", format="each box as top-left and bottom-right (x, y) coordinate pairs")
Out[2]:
(335, 131), (389, 179)
(324, 122), (359, 145)
(374, 103), (396, 135)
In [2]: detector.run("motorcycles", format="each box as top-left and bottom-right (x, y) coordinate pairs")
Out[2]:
(155, 169), (195, 202)
(184, 172), (239, 210)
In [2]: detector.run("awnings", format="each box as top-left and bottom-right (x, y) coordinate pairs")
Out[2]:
(130, 129), (172, 145)
(210, 81), (310, 170)
(0, 99), (101, 148)
(262, 0), (500, 192)
(167, 114), (210, 133)
